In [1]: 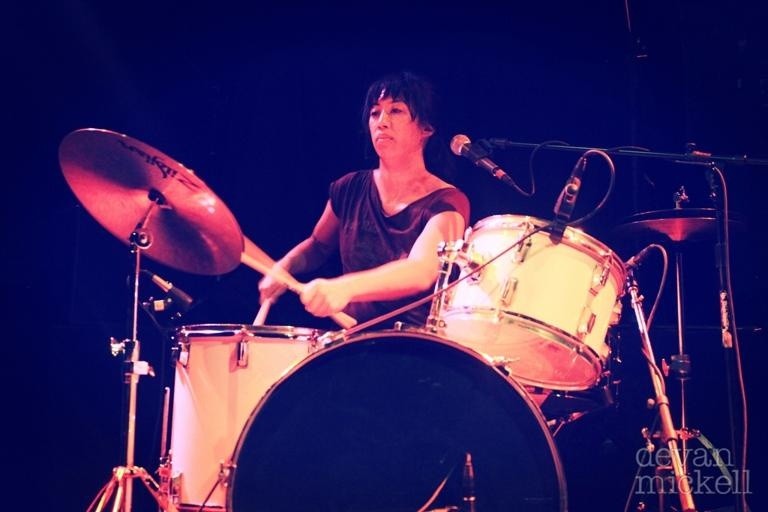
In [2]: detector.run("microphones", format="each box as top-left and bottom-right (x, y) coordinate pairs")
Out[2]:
(449, 133), (514, 186)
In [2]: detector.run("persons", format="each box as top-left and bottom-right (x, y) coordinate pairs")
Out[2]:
(253, 71), (475, 333)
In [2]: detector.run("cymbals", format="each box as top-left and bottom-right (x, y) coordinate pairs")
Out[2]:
(59, 127), (243, 275)
(615, 207), (719, 239)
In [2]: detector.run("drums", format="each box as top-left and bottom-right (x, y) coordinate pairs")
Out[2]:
(219, 322), (568, 512)
(425, 212), (628, 393)
(157, 323), (349, 512)
